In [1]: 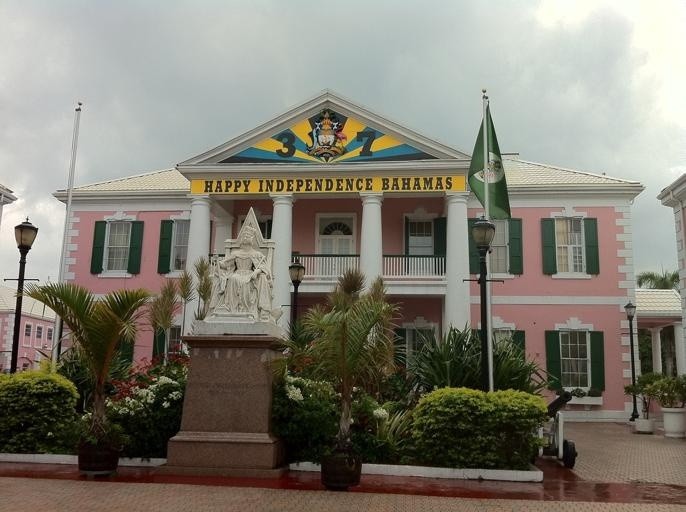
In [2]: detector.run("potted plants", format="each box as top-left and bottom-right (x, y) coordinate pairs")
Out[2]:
(267, 269), (404, 488)
(624, 372), (665, 433)
(14, 276), (149, 471)
(644, 374), (686, 438)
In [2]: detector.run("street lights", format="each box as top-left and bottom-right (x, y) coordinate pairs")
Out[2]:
(8, 216), (39, 372)
(472, 215), (498, 389)
(622, 301), (639, 416)
(285, 253), (308, 337)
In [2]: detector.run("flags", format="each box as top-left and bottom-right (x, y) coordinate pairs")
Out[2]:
(467, 100), (511, 220)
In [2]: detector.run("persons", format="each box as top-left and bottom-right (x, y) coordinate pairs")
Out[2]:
(213, 225), (267, 313)
